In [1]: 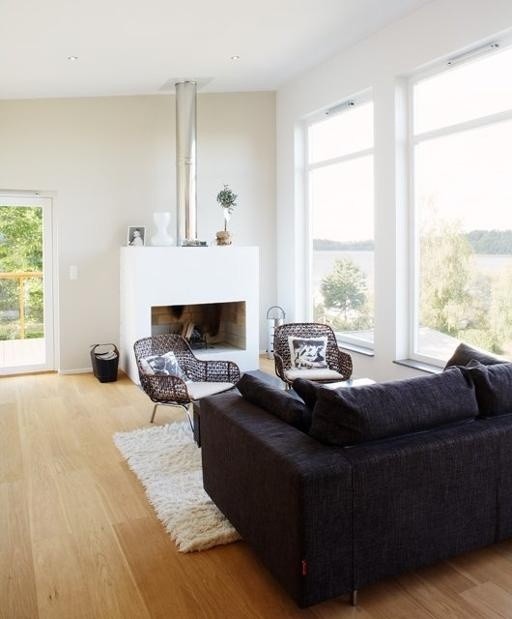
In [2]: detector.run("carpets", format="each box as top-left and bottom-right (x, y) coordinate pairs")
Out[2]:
(112, 419), (244, 554)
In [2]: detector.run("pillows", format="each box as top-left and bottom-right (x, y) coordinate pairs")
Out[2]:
(237, 363), (512, 446)
(139, 350), (194, 393)
(445, 350), (500, 367)
(287, 336), (330, 371)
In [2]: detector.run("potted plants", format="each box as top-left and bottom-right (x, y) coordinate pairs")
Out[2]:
(215, 184), (239, 247)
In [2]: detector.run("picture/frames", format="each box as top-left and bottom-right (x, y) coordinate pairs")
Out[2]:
(127, 226), (146, 247)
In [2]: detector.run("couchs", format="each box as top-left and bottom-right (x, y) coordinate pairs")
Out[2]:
(201, 343), (512, 610)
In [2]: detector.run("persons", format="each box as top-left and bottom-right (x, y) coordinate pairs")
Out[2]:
(128, 227), (144, 246)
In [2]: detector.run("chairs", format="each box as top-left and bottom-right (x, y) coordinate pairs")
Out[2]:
(271, 322), (352, 392)
(134, 334), (241, 432)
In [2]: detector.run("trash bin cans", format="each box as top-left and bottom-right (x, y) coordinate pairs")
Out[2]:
(90, 344), (119, 383)
(267, 306), (285, 359)
